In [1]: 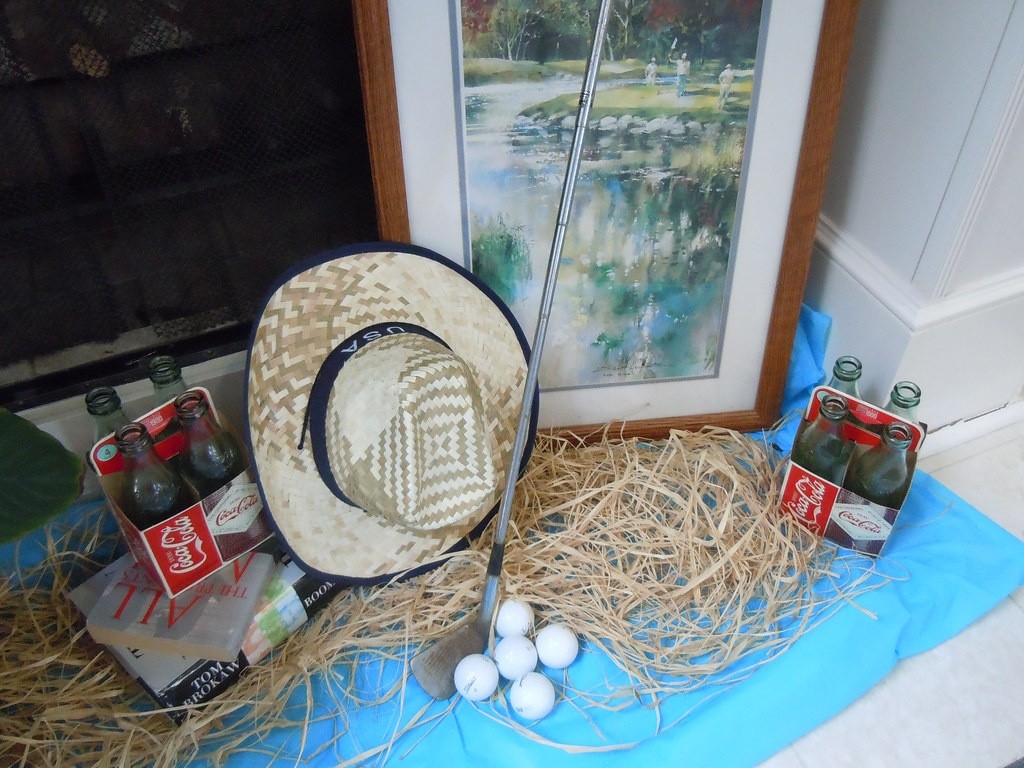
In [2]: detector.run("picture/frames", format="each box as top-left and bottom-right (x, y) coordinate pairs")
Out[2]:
(350, 1), (860, 452)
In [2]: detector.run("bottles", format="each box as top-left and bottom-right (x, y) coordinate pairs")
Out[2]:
(172, 390), (249, 498)
(146, 354), (190, 411)
(844, 421), (915, 509)
(826, 355), (863, 401)
(884, 380), (922, 425)
(792, 393), (852, 488)
(82, 384), (133, 445)
(111, 421), (194, 532)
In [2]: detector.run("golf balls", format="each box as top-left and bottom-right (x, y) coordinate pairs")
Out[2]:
(508, 672), (556, 720)
(454, 653), (500, 701)
(494, 636), (537, 681)
(493, 598), (536, 636)
(536, 624), (579, 669)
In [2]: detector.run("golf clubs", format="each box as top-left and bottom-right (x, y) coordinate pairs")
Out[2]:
(408, 0), (611, 701)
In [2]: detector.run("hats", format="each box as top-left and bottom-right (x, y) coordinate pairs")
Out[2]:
(241, 241), (540, 587)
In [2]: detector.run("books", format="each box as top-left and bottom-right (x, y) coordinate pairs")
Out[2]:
(67, 535), (354, 728)
